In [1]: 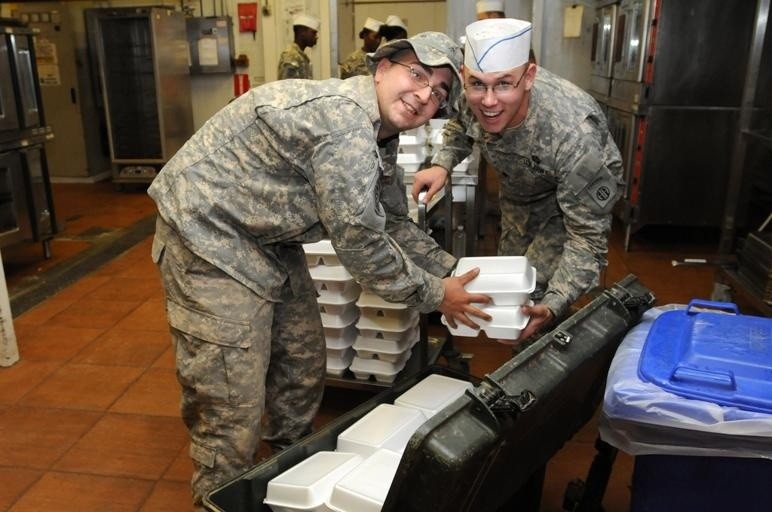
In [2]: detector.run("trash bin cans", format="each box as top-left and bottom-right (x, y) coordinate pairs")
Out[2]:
(603, 299), (772, 512)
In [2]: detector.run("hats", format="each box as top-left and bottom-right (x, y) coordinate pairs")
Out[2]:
(463, 19), (532, 73)
(366, 31), (465, 117)
(364, 15), (407, 33)
(293, 15), (320, 32)
(476, 0), (504, 13)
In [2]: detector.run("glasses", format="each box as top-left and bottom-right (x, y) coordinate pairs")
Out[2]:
(464, 69), (527, 94)
(391, 60), (449, 110)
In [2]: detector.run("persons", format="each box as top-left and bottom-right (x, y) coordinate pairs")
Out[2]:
(277, 0), (537, 80)
(412, 18), (626, 360)
(146, 29), (493, 512)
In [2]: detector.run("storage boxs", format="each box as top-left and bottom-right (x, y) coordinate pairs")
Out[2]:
(198, 272), (656, 512)
